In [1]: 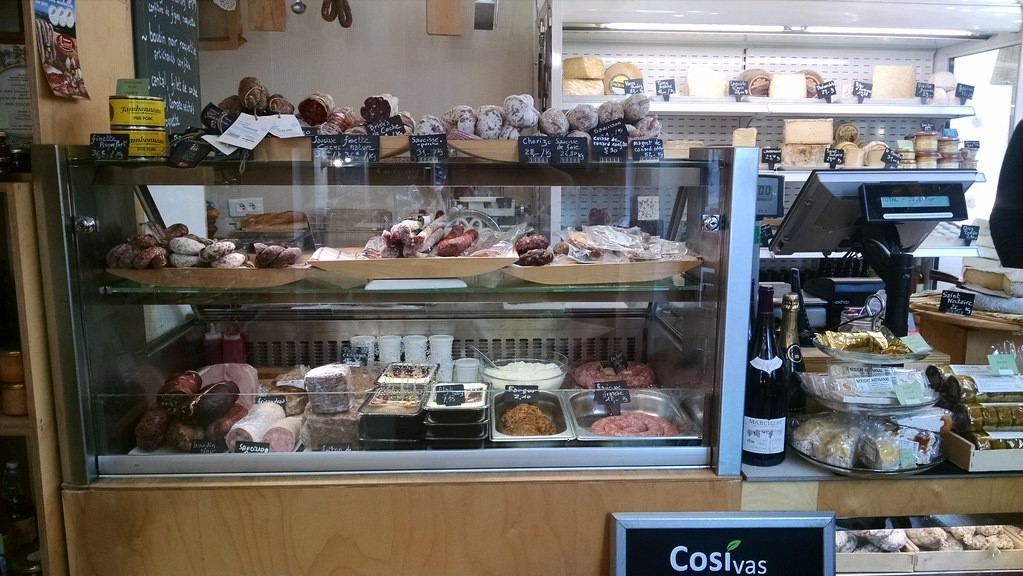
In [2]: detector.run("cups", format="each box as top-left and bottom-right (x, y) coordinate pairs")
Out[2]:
(429, 335), (453, 382)
(454, 357), (480, 383)
(350, 335), (375, 367)
(867, 148), (886, 167)
(377, 336), (402, 363)
(402, 335), (428, 364)
(845, 147), (864, 167)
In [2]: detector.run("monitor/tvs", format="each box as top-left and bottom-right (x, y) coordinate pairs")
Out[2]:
(769, 168), (979, 254)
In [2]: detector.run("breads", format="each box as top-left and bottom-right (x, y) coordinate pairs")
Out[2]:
(789, 410), (952, 470)
(929, 361), (1023, 451)
(925, 71), (965, 107)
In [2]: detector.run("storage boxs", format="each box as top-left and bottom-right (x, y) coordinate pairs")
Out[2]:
(836, 524), (1023, 576)
(358, 357), (491, 451)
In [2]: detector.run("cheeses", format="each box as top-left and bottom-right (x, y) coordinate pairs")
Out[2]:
(963, 266), (1023, 297)
(561, 56), (916, 100)
(660, 118), (889, 169)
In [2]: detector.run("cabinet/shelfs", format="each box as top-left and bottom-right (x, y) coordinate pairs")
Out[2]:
(558, 89), (986, 187)
(34, 142), (722, 486)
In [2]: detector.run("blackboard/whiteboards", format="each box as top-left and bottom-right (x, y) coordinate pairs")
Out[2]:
(594, 381), (632, 404)
(409, 133), (448, 162)
(235, 439), (271, 454)
(625, 72), (980, 169)
(517, 135), (589, 164)
(190, 438), (218, 455)
(505, 383), (539, 404)
(131, 0), (201, 144)
(589, 116), (629, 162)
(254, 395), (286, 413)
(436, 385), (466, 406)
(318, 442), (353, 453)
(89, 133), (130, 161)
(959, 223), (980, 241)
(365, 115), (407, 136)
(609, 348), (628, 376)
(340, 343), (369, 366)
(631, 139), (664, 161)
(310, 133), (380, 165)
(938, 288), (976, 316)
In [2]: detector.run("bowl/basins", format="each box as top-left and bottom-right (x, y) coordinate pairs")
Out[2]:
(478, 348), (568, 390)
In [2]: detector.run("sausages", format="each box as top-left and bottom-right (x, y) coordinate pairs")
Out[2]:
(132, 364), (302, 453)
(364, 212), (480, 258)
(213, 75), (663, 141)
(321, 0), (353, 28)
(834, 525), (1015, 554)
(574, 358), (680, 436)
(105, 221), (302, 269)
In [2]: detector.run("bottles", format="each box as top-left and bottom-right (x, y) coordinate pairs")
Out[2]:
(741, 282), (788, 467)
(839, 306), (882, 333)
(746, 279), (755, 359)
(914, 131), (978, 171)
(204, 323), (223, 367)
(223, 324), (243, 365)
(0, 460), (42, 576)
(775, 293), (805, 438)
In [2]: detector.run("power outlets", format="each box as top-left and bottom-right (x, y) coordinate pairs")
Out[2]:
(227, 198), (266, 217)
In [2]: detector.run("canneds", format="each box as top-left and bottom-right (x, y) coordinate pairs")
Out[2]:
(893, 130), (961, 170)
(0, 350), (28, 415)
(0, 131), (30, 182)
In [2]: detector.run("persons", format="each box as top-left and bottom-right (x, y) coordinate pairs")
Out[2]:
(988, 119), (1023, 268)
